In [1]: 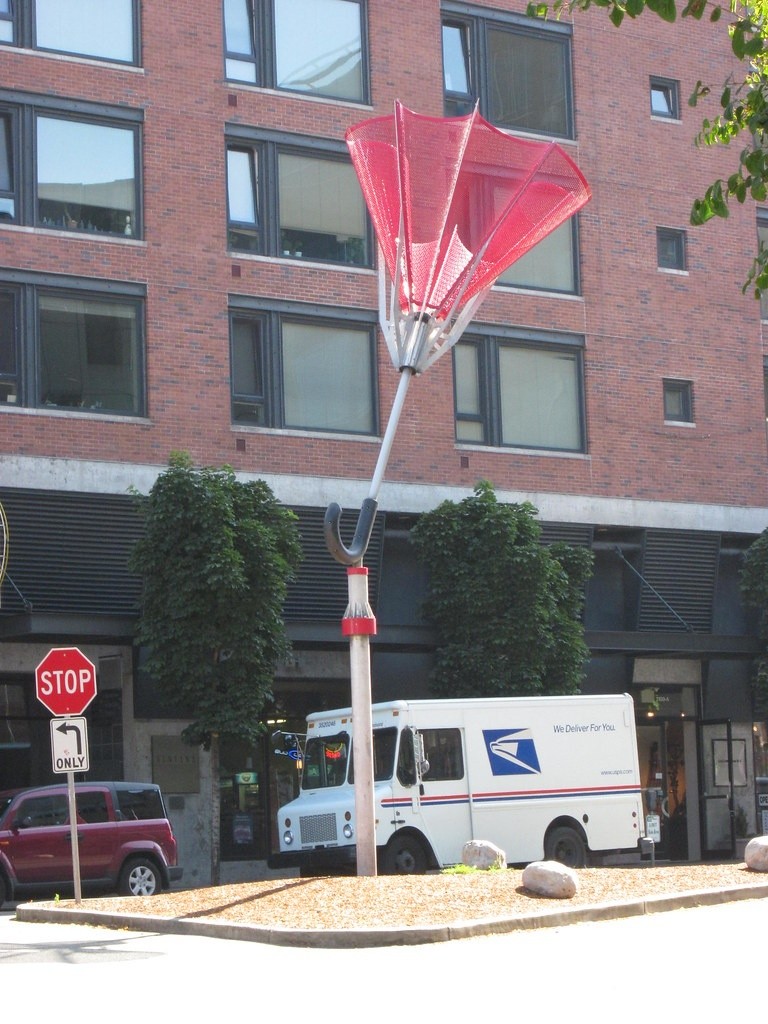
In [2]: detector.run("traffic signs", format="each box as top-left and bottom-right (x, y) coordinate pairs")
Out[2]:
(49, 717), (89, 774)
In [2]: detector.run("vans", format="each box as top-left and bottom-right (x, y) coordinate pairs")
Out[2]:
(271, 692), (655, 874)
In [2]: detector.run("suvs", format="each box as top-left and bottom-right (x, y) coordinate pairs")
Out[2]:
(0, 782), (183, 909)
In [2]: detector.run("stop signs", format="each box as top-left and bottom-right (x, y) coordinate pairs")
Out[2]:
(35, 647), (98, 717)
